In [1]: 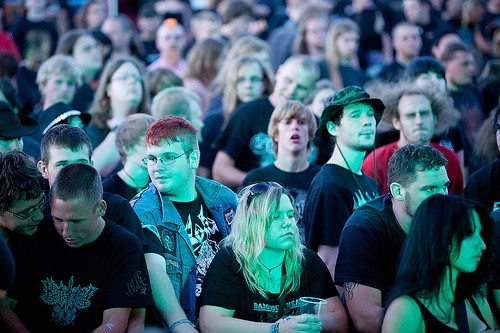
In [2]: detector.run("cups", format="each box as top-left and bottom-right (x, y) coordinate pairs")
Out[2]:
(300, 297), (328, 325)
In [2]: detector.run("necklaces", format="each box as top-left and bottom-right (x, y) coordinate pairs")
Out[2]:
(260, 260), (284, 287)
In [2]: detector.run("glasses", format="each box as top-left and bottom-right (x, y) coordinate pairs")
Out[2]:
(246, 181), (287, 207)
(2, 202), (50, 219)
(141, 148), (194, 166)
(111, 73), (144, 82)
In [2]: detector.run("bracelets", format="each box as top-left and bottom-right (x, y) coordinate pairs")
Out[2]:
(282, 315), (292, 321)
(168, 319), (196, 329)
(270, 323), (279, 333)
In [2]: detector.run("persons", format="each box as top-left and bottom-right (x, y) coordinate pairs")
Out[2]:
(243, 99), (324, 247)
(303, 86), (379, 282)
(360, 74), (464, 199)
(0, 0), (500, 333)
(86, 56), (151, 151)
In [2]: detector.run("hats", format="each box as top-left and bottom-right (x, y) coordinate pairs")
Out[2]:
(320, 86), (387, 127)
(39, 101), (92, 136)
